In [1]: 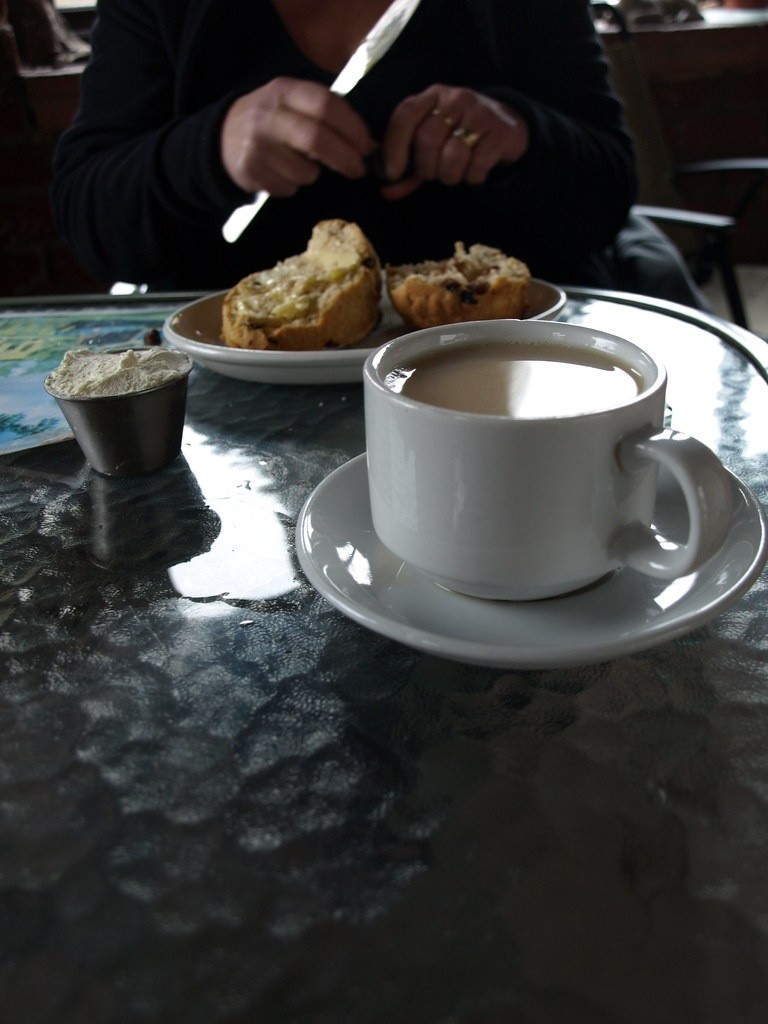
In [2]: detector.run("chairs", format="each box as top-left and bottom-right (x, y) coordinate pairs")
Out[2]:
(589, 4), (768, 328)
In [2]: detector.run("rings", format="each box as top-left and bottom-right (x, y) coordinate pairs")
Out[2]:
(453, 128), (479, 145)
(431, 109), (456, 127)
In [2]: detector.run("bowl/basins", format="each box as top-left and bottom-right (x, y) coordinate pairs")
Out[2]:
(44, 349), (194, 476)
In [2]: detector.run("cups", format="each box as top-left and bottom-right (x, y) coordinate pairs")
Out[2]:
(362, 319), (733, 601)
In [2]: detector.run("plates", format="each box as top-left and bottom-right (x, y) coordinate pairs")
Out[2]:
(292, 448), (767, 669)
(162, 276), (566, 384)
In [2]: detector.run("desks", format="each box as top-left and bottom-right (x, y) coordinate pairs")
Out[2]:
(1, 285), (768, 1024)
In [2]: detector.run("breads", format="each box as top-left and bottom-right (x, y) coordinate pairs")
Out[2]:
(220, 219), (381, 350)
(386, 243), (531, 328)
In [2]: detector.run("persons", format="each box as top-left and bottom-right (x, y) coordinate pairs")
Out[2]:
(42, 1), (637, 289)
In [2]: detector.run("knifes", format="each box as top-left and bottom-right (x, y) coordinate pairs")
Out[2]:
(221, 0), (422, 242)
